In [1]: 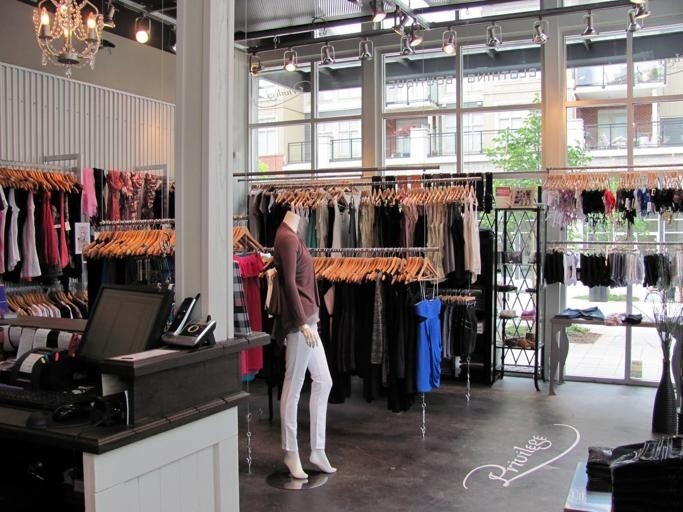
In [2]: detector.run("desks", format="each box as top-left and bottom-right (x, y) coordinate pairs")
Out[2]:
(564, 462), (613, 512)
(550, 317), (657, 395)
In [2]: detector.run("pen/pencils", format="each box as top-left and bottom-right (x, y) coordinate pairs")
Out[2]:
(40, 351), (65, 368)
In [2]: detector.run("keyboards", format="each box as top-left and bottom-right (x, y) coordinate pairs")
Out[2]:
(1, 387), (97, 411)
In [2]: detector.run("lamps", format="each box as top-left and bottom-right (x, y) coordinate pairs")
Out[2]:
(104, 0), (177, 54)
(319, 42), (339, 69)
(33, 0), (102, 76)
(580, 10), (601, 39)
(391, 12), (426, 58)
(623, 1), (653, 34)
(357, 36), (377, 62)
(366, 0), (388, 24)
(283, 47), (299, 74)
(249, 53), (265, 75)
(440, 28), (459, 55)
(484, 23), (505, 50)
(531, 17), (551, 46)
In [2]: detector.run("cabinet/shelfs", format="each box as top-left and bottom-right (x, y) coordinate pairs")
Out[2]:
(490, 208), (546, 391)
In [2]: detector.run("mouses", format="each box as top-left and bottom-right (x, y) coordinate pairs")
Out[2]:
(53, 402), (78, 422)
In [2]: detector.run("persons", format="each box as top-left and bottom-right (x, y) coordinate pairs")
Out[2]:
(275, 208), (339, 480)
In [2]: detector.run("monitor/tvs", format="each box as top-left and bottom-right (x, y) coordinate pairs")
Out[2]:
(74, 284), (176, 376)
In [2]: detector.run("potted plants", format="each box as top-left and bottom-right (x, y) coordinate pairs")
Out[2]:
(632, 288), (683, 436)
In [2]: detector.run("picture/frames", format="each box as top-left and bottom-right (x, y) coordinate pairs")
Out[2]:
(510, 188), (535, 208)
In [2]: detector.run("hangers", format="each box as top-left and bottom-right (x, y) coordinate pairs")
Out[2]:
(234, 217), (265, 252)
(82, 220), (176, 258)
(439, 289), (478, 305)
(542, 170), (682, 191)
(0, 162), (82, 194)
(6, 283), (88, 312)
(252, 178), (479, 208)
(546, 239), (683, 257)
(313, 248), (442, 285)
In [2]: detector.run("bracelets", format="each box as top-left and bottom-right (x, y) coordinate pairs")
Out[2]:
(298, 323), (310, 331)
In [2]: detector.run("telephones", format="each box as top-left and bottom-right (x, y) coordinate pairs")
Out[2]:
(161, 293), (216, 347)
